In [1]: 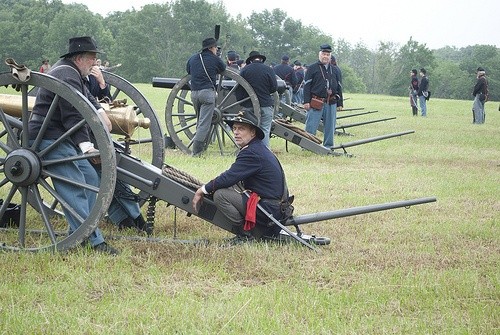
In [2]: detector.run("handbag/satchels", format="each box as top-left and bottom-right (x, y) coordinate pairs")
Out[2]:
(479, 94), (485, 103)
(311, 97), (323, 110)
(426, 90), (431, 100)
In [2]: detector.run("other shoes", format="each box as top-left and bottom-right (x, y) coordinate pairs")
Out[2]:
(92, 242), (118, 255)
(134, 213), (152, 234)
(228, 233), (255, 246)
(118, 218), (137, 228)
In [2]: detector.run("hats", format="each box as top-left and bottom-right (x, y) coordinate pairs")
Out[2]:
(282, 56), (289, 60)
(42, 58), (49, 63)
(224, 111), (264, 140)
(294, 60), (302, 65)
(59, 37), (99, 58)
(198, 38), (218, 52)
(320, 44), (332, 53)
(226, 50), (244, 69)
(478, 66), (484, 71)
(246, 51), (266, 65)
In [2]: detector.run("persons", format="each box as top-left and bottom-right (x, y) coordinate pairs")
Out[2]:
(419, 67), (429, 116)
(95, 57), (122, 96)
(26, 36), (155, 254)
(408, 69), (418, 116)
(304, 43), (344, 150)
(218, 46), (338, 127)
(188, 112), (291, 246)
(38, 58), (51, 76)
(235, 50), (276, 154)
(471, 67), (488, 124)
(77, 54), (153, 236)
(186, 37), (227, 156)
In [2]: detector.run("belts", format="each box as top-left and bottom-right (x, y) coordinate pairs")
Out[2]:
(313, 94), (335, 101)
